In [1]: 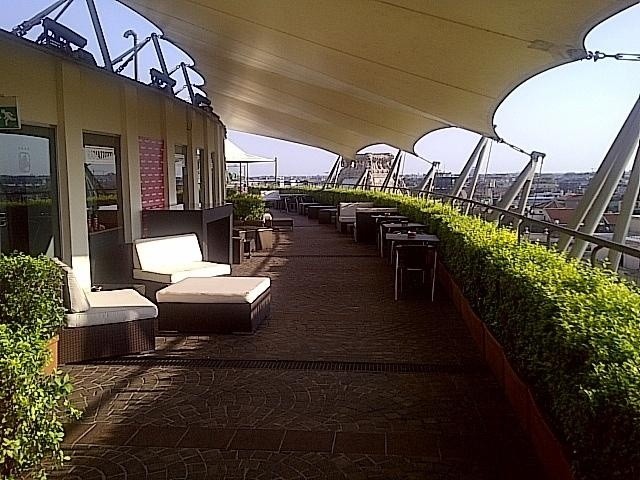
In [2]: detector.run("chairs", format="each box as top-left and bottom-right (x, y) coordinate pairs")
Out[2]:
(132, 233), (231, 305)
(50, 257), (158, 366)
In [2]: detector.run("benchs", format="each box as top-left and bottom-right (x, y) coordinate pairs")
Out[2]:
(156, 276), (271, 335)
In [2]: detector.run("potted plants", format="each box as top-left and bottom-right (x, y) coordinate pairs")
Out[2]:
(0, 250), (83, 480)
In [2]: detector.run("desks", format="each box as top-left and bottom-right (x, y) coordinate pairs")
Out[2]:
(278, 193), (440, 302)
